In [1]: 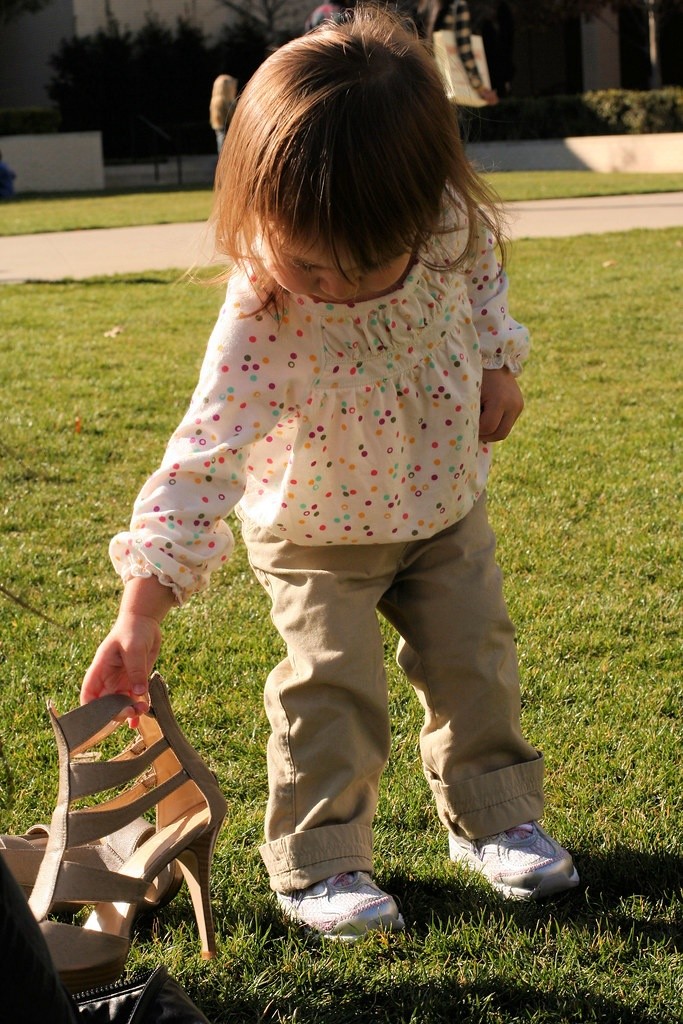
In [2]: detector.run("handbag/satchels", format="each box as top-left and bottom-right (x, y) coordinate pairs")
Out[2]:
(432, 30), (499, 106)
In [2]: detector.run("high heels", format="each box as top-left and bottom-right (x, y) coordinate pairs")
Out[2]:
(0, 671), (228, 998)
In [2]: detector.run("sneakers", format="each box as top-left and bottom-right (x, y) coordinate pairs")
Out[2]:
(448, 821), (579, 903)
(276, 871), (406, 946)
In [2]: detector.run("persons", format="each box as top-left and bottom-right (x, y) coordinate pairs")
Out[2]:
(80, 7), (579, 943)
